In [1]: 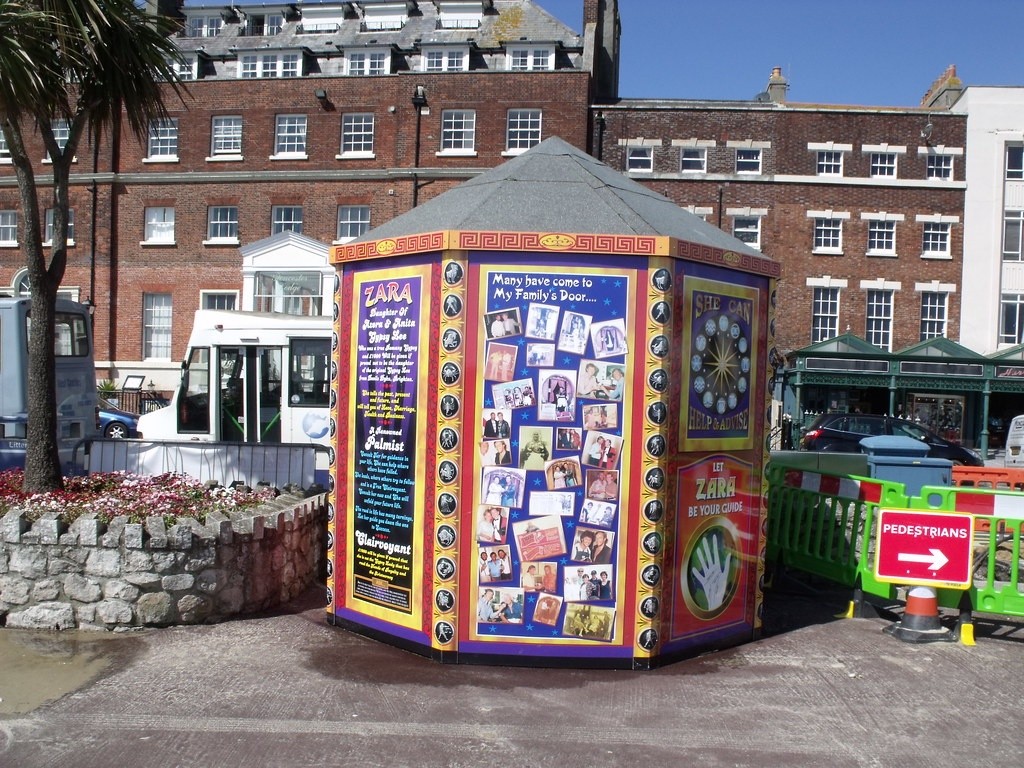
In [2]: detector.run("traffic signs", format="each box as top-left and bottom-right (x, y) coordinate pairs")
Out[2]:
(875, 509), (974, 585)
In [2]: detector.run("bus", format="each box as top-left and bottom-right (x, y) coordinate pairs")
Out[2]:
(135, 306), (336, 489)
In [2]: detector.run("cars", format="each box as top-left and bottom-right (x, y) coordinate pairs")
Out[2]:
(803, 413), (988, 467)
(96, 397), (138, 438)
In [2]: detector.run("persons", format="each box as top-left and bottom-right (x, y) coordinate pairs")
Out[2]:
(478, 589), (523, 623)
(479, 441), (510, 465)
(575, 530), (612, 563)
(524, 521), (540, 533)
(568, 568), (612, 600)
(588, 436), (616, 469)
(537, 599), (559, 623)
(522, 564), (556, 592)
(589, 471), (617, 500)
(489, 308), (624, 417)
(478, 508), (507, 543)
(554, 463), (577, 488)
(558, 429), (580, 449)
(479, 549), (511, 582)
(583, 502), (614, 525)
(485, 412), (510, 438)
(522, 432), (549, 469)
(485, 475), (516, 507)
(572, 611), (612, 639)
(586, 409), (608, 429)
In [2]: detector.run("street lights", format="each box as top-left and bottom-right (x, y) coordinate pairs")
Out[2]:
(411, 90), (429, 211)
